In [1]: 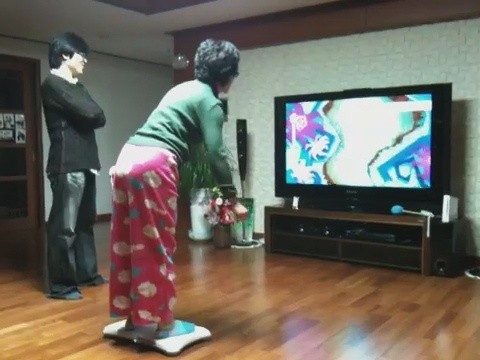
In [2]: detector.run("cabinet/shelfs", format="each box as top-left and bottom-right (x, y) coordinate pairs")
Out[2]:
(262, 203), (433, 276)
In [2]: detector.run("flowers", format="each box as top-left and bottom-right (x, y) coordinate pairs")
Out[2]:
(201, 198), (237, 226)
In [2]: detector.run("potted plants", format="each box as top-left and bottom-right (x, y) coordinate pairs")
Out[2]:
(176, 142), (237, 243)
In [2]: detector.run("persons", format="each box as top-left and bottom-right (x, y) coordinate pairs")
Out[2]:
(39, 31), (110, 301)
(108, 38), (248, 340)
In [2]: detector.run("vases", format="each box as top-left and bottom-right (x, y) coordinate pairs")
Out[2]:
(213, 222), (229, 250)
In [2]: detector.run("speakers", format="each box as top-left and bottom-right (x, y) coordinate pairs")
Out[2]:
(430, 215), (467, 278)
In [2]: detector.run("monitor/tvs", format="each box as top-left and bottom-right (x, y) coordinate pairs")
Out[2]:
(274, 82), (452, 214)
